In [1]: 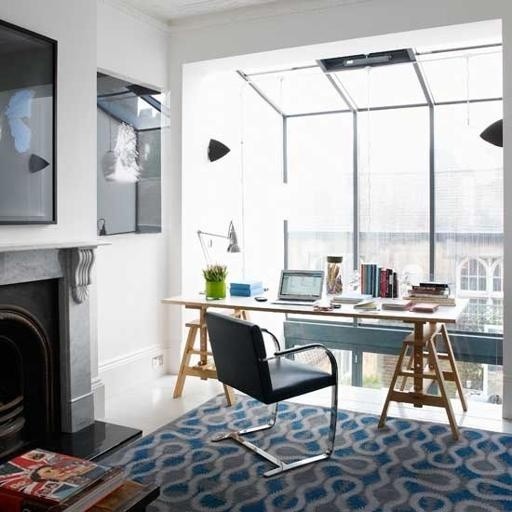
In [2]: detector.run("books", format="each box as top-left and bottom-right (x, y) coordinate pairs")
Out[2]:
(332, 262), (457, 312)
(230, 288), (264, 297)
(0, 395), (126, 511)
(230, 280), (263, 289)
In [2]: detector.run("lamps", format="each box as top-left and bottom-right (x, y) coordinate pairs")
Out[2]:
(197, 220), (240, 264)
(103, 95), (124, 181)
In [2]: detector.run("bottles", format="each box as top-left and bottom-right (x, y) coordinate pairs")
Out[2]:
(325, 255), (344, 295)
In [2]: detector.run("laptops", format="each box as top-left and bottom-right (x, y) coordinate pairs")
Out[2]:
(271, 270), (325, 305)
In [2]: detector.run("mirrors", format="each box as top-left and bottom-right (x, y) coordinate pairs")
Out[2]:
(95, 69), (162, 234)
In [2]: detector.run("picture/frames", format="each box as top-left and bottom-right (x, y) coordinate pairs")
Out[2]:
(0, 20), (57, 225)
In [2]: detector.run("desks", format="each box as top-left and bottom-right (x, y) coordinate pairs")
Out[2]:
(86, 480), (160, 512)
(160, 295), (470, 440)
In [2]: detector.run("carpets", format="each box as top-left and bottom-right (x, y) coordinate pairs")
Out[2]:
(95, 393), (512, 512)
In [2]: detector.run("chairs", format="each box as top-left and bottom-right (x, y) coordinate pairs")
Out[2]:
(204, 312), (338, 478)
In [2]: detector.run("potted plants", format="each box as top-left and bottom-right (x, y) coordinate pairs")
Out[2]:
(203, 261), (229, 299)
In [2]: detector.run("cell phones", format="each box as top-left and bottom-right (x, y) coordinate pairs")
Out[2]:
(255, 297), (267, 302)
